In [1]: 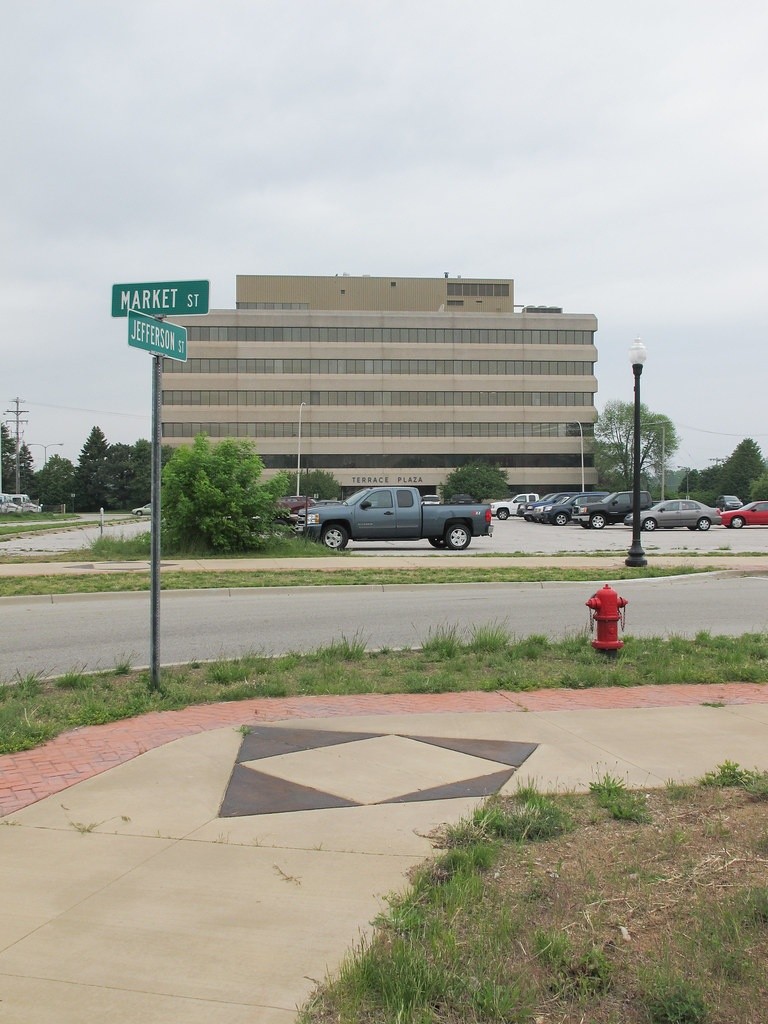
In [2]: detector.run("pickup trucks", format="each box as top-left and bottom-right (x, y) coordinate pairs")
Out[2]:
(571, 491), (664, 530)
(292, 487), (496, 553)
(489, 493), (540, 520)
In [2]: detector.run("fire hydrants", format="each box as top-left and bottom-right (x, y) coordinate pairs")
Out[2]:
(586, 585), (632, 655)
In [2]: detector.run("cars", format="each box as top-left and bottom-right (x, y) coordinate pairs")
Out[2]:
(717, 495), (743, 510)
(272, 495), (315, 532)
(718, 501), (768, 528)
(0, 493), (42, 514)
(623, 499), (722, 531)
(132, 503), (152, 516)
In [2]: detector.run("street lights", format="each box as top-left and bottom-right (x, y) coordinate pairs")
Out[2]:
(626, 335), (650, 567)
(27, 443), (63, 466)
(295, 401), (307, 496)
(677, 465), (691, 501)
(657, 417), (666, 501)
(574, 418), (584, 492)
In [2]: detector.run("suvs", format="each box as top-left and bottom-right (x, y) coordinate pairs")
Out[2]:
(515, 491), (610, 526)
